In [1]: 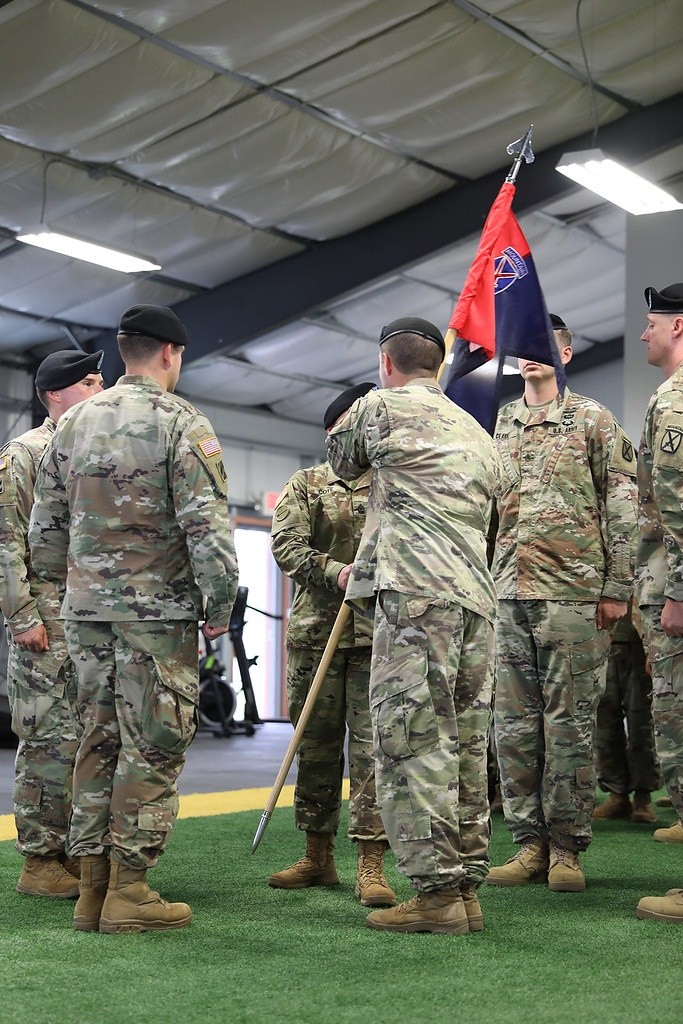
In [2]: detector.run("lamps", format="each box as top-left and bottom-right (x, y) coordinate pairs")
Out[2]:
(16, 157), (164, 274)
(554, 0), (683, 215)
(443, 296), (520, 375)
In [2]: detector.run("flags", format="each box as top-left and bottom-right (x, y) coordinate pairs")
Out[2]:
(446, 182), (567, 397)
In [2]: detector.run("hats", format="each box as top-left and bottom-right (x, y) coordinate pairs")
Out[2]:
(549, 313), (568, 330)
(380, 317), (445, 363)
(324, 382), (379, 430)
(35, 350), (105, 391)
(118, 304), (189, 345)
(644, 283), (683, 314)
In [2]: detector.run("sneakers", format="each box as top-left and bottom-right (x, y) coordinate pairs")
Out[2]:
(460, 885), (484, 931)
(16, 855), (81, 898)
(637, 888), (683, 923)
(548, 839), (586, 891)
(656, 797), (674, 807)
(366, 884), (470, 934)
(629, 793), (657, 823)
(654, 818), (683, 843)
(486, 836), (549, 886)
(591, 794), (632, 821)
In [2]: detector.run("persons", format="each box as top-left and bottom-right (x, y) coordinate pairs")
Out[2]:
(0, 349), (108, 907)
(271, 311), (663, 937)
(629, 281), (683, 924)
(28, 305), (240, 934)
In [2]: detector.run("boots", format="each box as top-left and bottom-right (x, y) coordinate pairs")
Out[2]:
(74, 856), (110, 931)
(354, 841), (400, 906)
(98, 856), (192, 933)
(269, 833), (340, 889)
